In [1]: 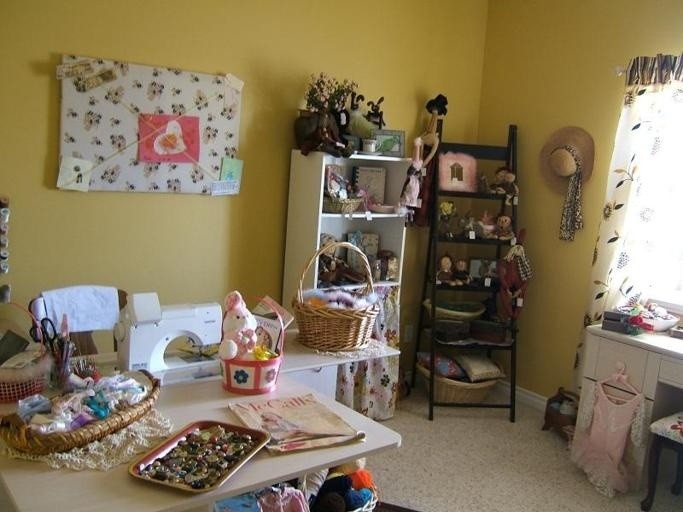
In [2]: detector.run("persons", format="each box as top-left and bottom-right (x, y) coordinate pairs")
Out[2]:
(257, 410), (352, 443)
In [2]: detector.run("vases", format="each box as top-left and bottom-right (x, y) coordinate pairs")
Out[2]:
(294, 109), (352, 158)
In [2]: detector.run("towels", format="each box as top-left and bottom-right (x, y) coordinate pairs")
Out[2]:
(41, 284), (121, 337)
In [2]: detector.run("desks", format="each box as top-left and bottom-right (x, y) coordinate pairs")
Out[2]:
(0, 374), (401, 512)
(570, 324), (682, 494)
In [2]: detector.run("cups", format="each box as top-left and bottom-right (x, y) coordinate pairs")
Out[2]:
(361, 139), (382, 152)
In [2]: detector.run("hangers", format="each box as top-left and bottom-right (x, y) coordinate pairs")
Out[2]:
(599, 366), (638, 403)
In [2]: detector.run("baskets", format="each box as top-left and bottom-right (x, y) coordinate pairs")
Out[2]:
(415, 360), (499, 407)
(423, 297), (486, 329)
(352, 475), (380, 511)
(294, 241), (380, 351)
(1, 301), (49, 405)
(322, 198), (363, 214)
(2, 370), (162, 456)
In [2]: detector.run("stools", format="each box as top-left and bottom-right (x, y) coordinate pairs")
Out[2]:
(640, 412), (682, 511)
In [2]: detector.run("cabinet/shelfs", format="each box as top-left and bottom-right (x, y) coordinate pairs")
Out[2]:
(282, 148), (413, 422)
(414, 118), (518, 421)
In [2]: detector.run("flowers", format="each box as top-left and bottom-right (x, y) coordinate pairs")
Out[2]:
(303, 71), (359, 112)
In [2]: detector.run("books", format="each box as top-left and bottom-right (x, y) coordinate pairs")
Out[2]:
(252, 294), (295, 330)
(253, 314), (282, 352)
(230, 392), (365, 456)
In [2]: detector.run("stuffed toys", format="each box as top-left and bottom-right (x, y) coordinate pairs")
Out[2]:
(412, 93), (449, 166)
(436, 250), (499, 286)
(478, 210), (498, 238)
(498, 215), (515, 240)
(324, 165), (373, 221)
(499, 228), (531, 322)
(219, 289), (258, 362)
(440, 201), (458, 239)
(399, 137), (423, 227)
(504, 237), (533, 281)
(318, 232), (399, 289)
(459, 209), (478, 239)
(479, 167), (519, 206)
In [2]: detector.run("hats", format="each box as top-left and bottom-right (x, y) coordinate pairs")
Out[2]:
(538, 126), (595, 241)
(496, 166), (505, 172)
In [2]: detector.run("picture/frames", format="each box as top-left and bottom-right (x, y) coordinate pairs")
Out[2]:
(369, 129), (404, 158)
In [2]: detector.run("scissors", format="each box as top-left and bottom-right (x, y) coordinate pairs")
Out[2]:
(28, 318), (57, 353)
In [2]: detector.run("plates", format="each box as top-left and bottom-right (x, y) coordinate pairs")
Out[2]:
(129, 420), (272, 494)
(356, 151), (383, 156)
(367, 203), (398, 213)
